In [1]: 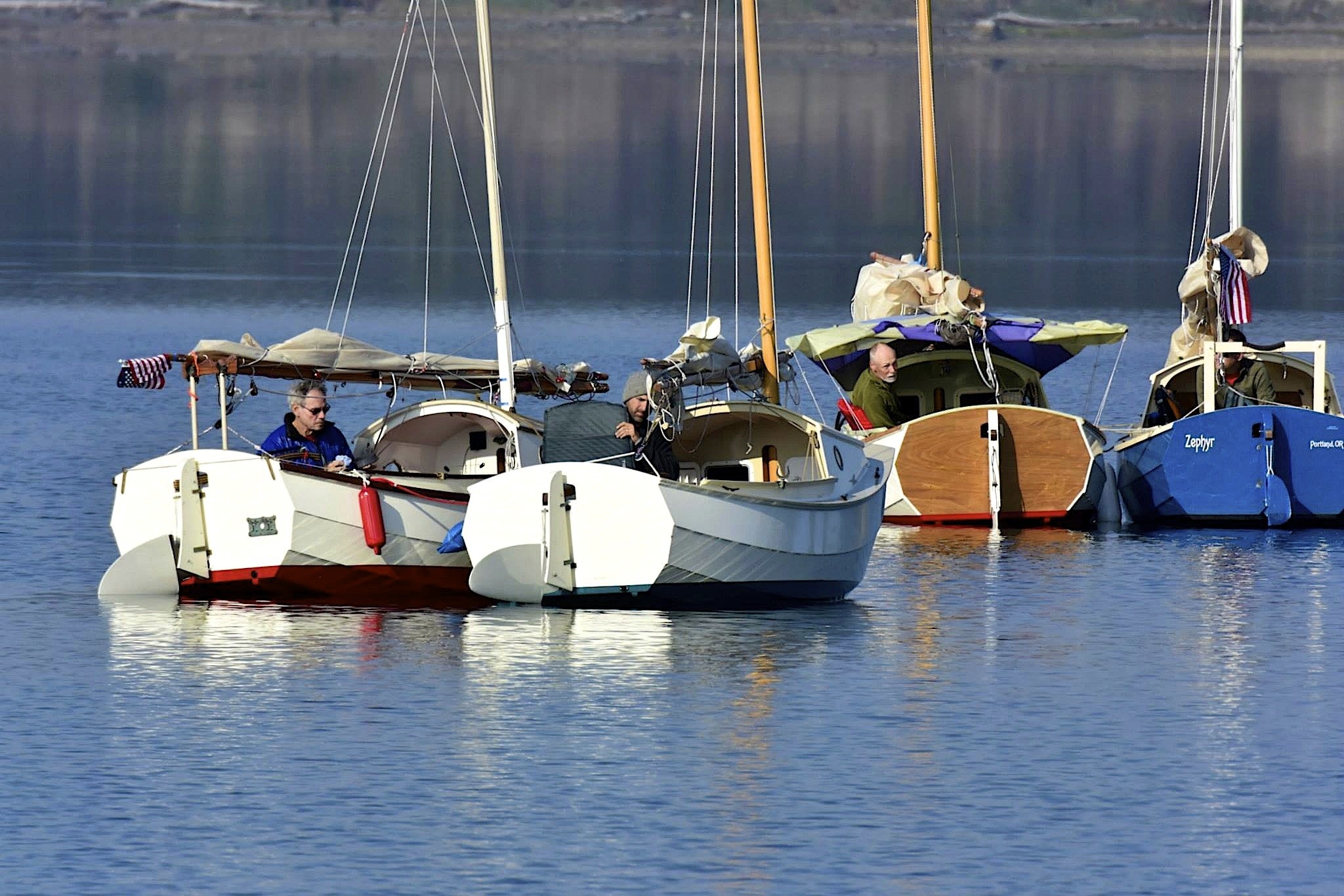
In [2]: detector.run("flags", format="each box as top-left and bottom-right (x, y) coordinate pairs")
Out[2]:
(115, 353), (172, 389)
(1217, 244), (1252, 325)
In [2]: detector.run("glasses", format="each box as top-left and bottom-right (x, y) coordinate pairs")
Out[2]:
(1215, 352), (1236, 359)
(298, 404), (331, 415)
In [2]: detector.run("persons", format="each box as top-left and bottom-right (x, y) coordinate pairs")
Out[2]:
(256, 380), (358, 473)
(1196, 329), (1276, 415)
(615, 369), (681, 480)
(850, 342), (909, 429)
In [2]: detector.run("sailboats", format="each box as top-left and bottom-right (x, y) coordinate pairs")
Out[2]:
(96, 1), (896, 612)
(1091, 0), (1344, 531)
(782, 1), (1132, 533)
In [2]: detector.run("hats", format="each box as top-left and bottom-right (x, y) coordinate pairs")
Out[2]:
(622, 372), (649, 403)
